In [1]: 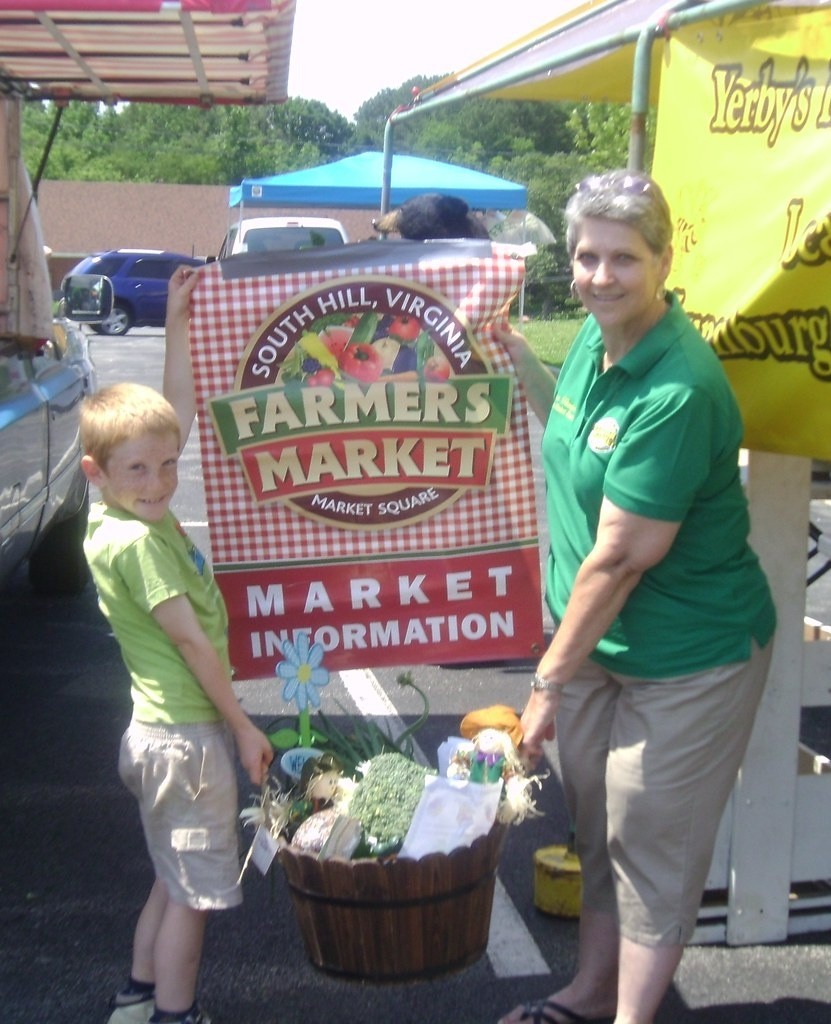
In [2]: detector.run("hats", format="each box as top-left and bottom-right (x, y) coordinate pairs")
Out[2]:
(374, 193), (488, 241)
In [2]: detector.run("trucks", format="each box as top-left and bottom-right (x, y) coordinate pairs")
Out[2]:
(0, 0), (298, 608)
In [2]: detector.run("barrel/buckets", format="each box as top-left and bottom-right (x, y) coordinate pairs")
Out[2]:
(257, 751), (528, 985)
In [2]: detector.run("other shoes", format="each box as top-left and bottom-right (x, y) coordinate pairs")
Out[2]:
(148, 998), (216, 1024)
(105, 990), (156, 1024)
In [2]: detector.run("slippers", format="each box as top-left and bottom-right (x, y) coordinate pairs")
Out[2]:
(519, 995), (616, 1024)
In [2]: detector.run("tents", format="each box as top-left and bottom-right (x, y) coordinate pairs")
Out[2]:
(225, 148), (529, 330)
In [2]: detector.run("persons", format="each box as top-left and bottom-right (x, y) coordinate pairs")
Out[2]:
(79, 264), (274, 1024)
(491, 174), (777, 1024)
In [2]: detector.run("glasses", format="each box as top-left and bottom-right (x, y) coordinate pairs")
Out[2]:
(574, 173), (669, 223)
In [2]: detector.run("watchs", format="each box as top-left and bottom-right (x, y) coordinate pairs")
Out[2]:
(531, 673), (562, 692)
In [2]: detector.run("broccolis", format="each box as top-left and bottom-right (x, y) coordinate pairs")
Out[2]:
(341, 750), (435, 842)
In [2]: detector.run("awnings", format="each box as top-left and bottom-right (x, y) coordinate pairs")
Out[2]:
(0, 1), (298, 264)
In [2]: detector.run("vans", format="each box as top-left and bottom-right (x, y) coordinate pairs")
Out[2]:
(206, 216), (349, 264)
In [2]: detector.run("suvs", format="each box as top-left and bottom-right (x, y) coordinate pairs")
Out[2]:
(61, 249), (202, 336)
